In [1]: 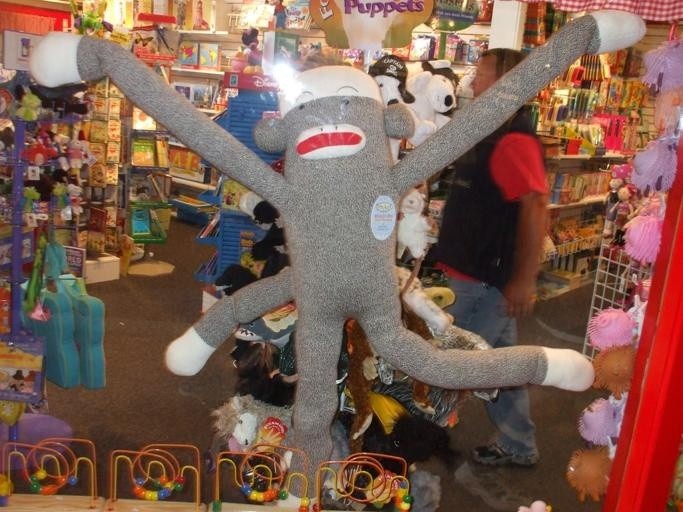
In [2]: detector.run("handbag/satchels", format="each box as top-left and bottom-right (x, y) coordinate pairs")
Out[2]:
(18, 239), (107, 390)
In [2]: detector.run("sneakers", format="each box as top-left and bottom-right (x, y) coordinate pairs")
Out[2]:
(471, 439), (541, 468)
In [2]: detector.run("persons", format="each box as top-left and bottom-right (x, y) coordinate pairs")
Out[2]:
(431, 46), (552, 470)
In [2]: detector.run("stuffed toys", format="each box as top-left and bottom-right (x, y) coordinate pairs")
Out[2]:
(25, 10), (648, 509)
(0, 69), (98, 226)
(205, 51), (501, 510)
(562, 35), (682, 501)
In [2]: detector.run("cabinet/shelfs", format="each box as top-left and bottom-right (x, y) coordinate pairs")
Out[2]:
(164, 27), (229, 191)
(534, 153), (635, 303)
